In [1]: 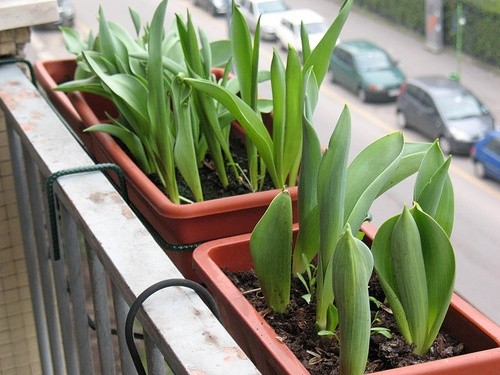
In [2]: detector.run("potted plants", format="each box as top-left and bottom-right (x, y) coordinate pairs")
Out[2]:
(192, 101), (500, 375)
(32, 1), (327, 293)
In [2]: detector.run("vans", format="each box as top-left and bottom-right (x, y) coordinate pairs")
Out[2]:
(330, 39), (406, 102)
(395, 75), (495, 155)
(274, 8), (330, 66)
(235, 0), (289, 42)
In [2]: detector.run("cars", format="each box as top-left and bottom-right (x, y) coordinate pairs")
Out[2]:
(470, 130), (500, 183)
(192, 0), (240, 16)
